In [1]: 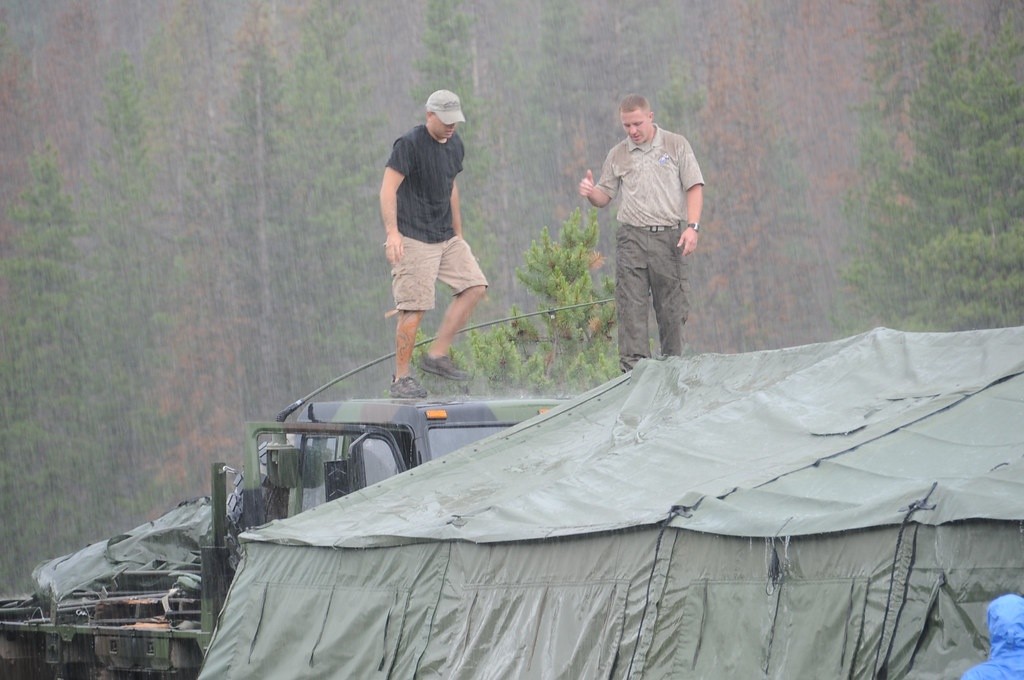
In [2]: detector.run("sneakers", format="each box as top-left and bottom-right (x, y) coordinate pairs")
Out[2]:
(391, 374), (427, 398)
(420, 352), (467, 380)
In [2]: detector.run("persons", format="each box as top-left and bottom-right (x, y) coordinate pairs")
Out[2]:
(578, 94), (707, 375)
(379, 88), (490, 399)
(960, 594), (1024, 680)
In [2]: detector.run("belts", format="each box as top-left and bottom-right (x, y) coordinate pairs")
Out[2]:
(621, 222), (679, 233)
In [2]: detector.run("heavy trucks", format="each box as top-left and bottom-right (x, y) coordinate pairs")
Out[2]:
(0, 395), (570, 680)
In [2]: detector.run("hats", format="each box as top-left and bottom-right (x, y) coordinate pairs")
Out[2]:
(426, 89), (465, 124)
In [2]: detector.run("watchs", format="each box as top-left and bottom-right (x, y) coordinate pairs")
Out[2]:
(688, 223), (700, 232)
